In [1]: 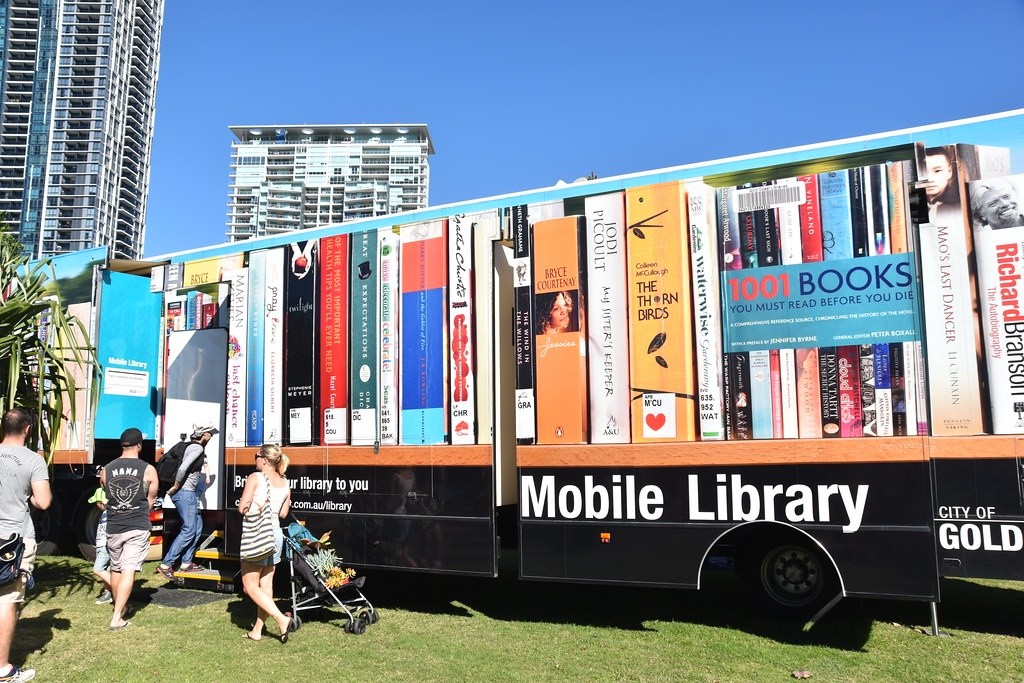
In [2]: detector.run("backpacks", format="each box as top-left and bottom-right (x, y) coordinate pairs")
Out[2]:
(155, 442), (200, 490)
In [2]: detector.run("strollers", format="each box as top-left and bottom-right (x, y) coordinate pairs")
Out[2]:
(280, 506), (379, 635)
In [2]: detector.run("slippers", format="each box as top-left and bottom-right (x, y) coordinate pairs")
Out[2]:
(281, 618), (293, 644)
(121, 606), (133, 618)
(108, 622), (131, 630)
(241, 632), (262, 641)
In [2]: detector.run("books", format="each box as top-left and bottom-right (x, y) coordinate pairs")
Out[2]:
(160, 140), (1024, 443)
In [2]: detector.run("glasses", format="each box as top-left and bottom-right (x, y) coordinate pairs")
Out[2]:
(255, 454), (265, 461)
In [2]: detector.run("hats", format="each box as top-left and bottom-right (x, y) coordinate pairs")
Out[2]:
(191, 420), (220, 435)
(120, 428), (147, 447)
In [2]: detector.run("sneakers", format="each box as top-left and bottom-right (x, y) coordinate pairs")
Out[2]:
(180, 562), (207, 572)
(157, 566), (179, 582)
(95, 591), (113, 605)
(0, 665), (36, 683)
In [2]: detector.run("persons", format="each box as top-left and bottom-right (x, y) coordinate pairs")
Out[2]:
(100, 427), (159, 630)
(385, 466), (417, 566)
(970, 180), (1024, 229)
(156, 422), (219, 581)
(87, 488), (113, 604)
(922, 145), (958, 216)
(0, 406), (52, 682)
(238, 444), (293, 643)
(535, 290), (572, 334)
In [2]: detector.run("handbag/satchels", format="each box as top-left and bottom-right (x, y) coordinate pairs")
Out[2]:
(239, 471), (277, 561)
(0, 532), (25, 587)
